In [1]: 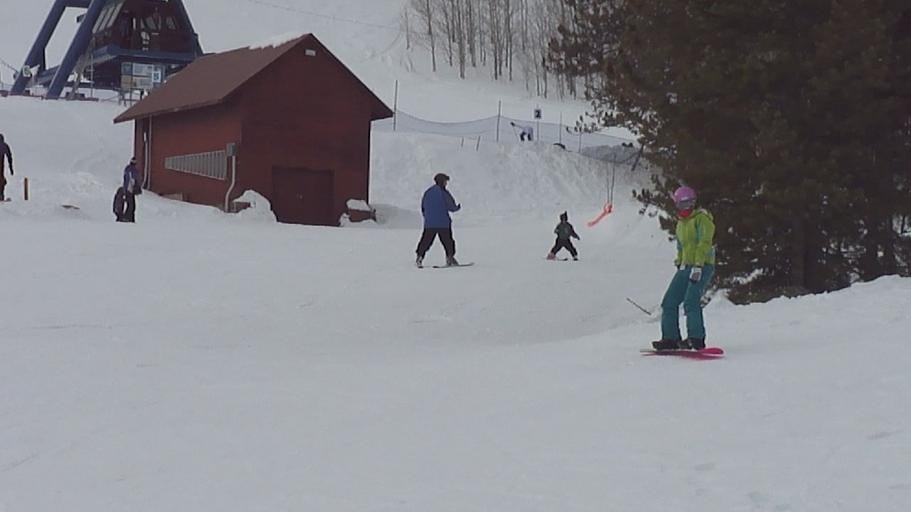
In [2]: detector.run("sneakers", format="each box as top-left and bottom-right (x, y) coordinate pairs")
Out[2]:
(416, 256), (462, 268)
(652, 338), (706, 351)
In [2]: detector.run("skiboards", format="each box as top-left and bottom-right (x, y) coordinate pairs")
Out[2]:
(416, 259), (474, 268)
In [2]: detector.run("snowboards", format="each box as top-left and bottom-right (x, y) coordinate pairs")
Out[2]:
(638, 346), (725, 359)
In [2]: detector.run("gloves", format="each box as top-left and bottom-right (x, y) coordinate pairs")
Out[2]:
(690, 266), (703, 283)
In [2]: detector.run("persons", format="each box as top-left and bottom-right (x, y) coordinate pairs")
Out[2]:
(416, 172), (463, 265)
(510, 121), (534, 141)
(547, 210), (581, 261)
(121, 156), (142, 222)
(1, 133), (15, 201)
(651, 186), (718, 348)
(113, 186), (127, 223)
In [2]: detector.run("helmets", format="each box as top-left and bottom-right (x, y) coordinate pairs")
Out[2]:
(673, 186), (698, 204)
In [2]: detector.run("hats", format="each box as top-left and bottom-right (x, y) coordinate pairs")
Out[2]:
(434, 173), (450, 186)
